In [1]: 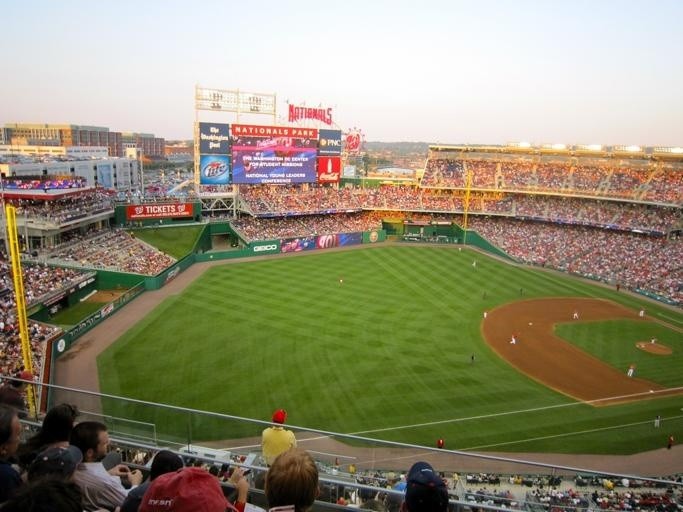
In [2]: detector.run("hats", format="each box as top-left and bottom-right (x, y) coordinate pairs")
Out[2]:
(407, 461), (448, 512)
(151, 451), (183, 478)
(27, 445), (83, 485)
(273, 410), (287, 423)
(138, 467), (239, 512)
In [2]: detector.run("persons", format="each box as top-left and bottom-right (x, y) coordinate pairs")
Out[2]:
(421, 159), (682, 306)
(234, 169), (422, 241)
(0, 176), (182, 410)
(0, 403), (682, 512)
(471, 288), (658, 376)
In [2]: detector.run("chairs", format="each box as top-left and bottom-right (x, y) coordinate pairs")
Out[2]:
(34, 230), (175, 276)
